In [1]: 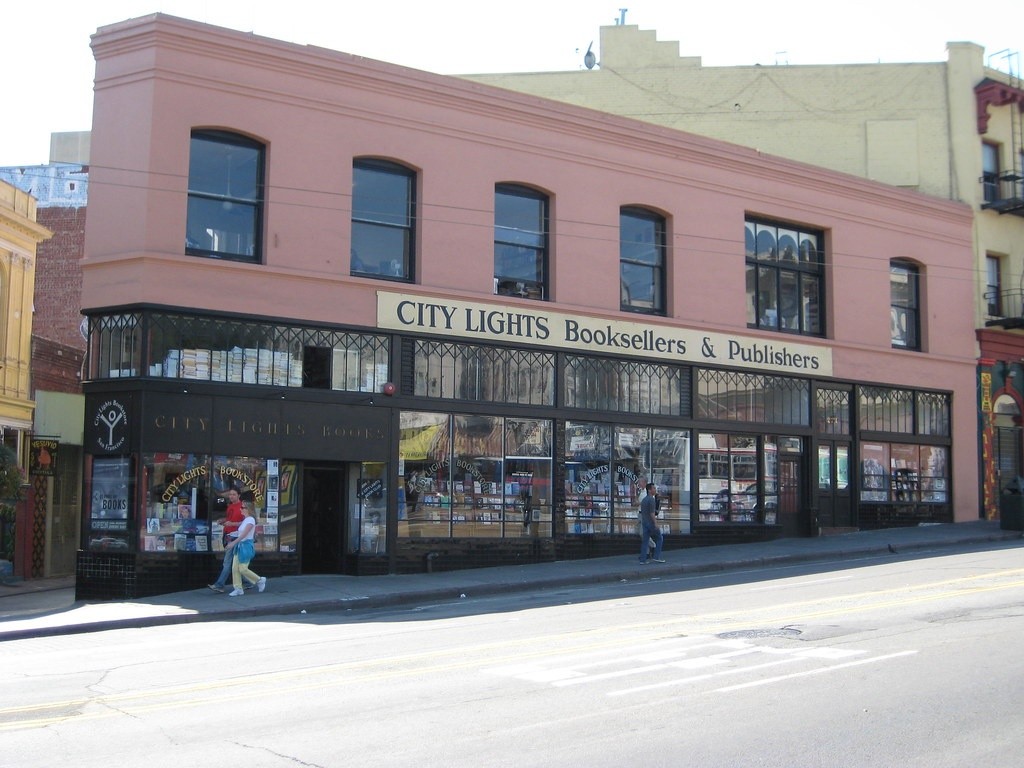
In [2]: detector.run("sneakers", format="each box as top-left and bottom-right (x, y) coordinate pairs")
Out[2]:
(229, 588), (244, 597)
(256, 577), (266, 592)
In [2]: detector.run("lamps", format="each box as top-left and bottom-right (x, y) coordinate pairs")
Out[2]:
(222, 154), (233, 213)
(1003, 369), (1017, 378)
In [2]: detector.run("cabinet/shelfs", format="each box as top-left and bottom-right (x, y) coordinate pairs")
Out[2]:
(405, 492), (552, 537)
(565, 493), (672, 524)
(882, 452), (934, 499)
(653, 463), (680, 534)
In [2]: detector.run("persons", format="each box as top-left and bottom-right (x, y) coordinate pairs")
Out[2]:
(525, 484), (541, 535)
(207, 486), (253, 593)
(354, 511), (381, 552)
(631, 475), (666, 564)
(224, 500), (266, 596)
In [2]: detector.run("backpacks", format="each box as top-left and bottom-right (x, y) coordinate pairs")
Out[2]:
(654, 494), (663, 515)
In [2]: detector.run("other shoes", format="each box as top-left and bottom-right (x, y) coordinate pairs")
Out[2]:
(640, 559), (650, 565)
(208, 582), (225, 592)
(653, 556), (665, 563)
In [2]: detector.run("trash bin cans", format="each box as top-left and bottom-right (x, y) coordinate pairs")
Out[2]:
(1000, 474), (1024, 530)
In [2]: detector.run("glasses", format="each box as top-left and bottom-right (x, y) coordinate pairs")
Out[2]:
(240, 506), (247, 510)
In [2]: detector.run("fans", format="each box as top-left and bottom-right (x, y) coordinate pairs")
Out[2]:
(391, 260), (400, 277)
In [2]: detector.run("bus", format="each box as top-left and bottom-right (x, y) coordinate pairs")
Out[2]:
(693, 446), (851, 523)
